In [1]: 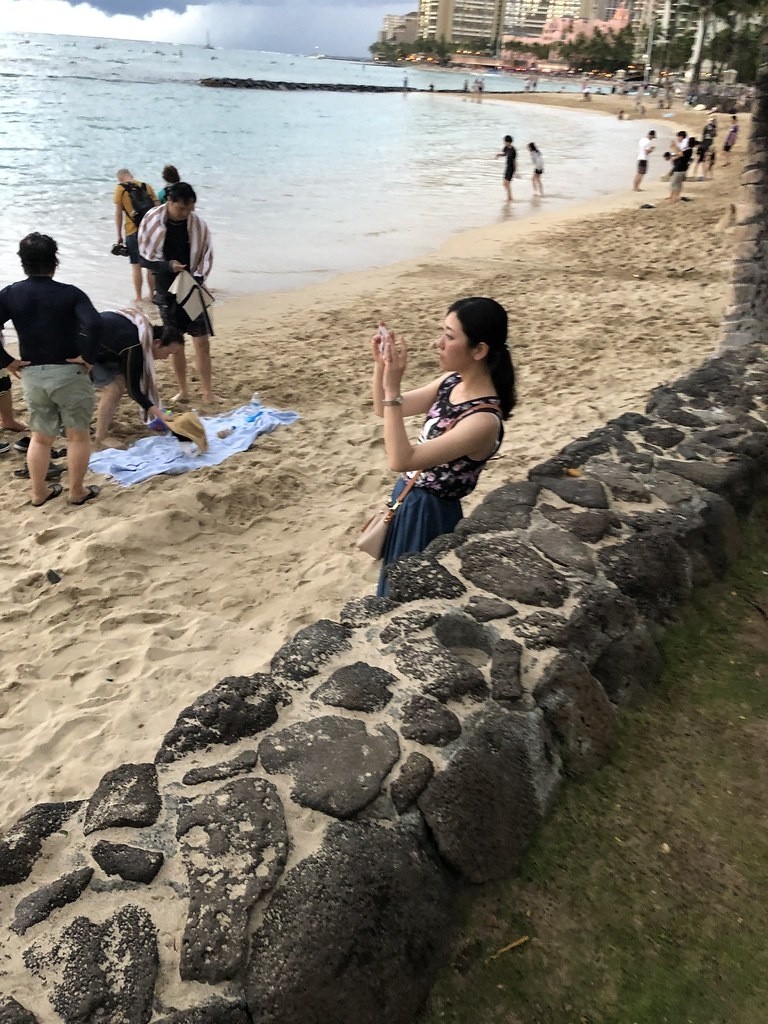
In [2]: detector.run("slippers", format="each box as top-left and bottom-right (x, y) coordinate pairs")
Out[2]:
(120, 247), (130, 257)
(110, 245), (123, 254)
(72, 484), (100, 505)
(31, 484), (63, 507)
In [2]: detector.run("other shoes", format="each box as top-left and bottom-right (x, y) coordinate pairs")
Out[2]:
(12, 436), (34, 451)
(24, 460), (68, 473)
(0, 441), (11, 453)
(14, 463), (60, 480)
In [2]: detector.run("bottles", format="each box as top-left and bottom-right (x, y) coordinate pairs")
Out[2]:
(250, 393), (260, 415)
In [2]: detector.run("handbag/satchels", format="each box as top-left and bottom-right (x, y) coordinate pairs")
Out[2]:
(151, 291), (181, 325)
(167, 269), (215, 326)
(355, 503), (392, 562)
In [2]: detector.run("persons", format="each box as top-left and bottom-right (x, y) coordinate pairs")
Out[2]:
(557, 86), (565, 93)
(114, 169), (161, 303)
(137, 181), (240, 404)
(722, 116), (738, 166)
(80, 308), (186, 449)
(663, 115), (718, 202)
(464, 78), (484, 93)
(497, 135), (516, 199)
(524, 77), (537, 93)
(527, 142), (544, 195)
(157, 165), (181, 204)
(429, 83), (434, 91)
(403, 71), (408, 92)
(0, 232), (104, 506)
(370, 296), (518, 595)
(0, 335), (29, 432)
(580, 74), (672, 121)
(634, 130), (658, 192)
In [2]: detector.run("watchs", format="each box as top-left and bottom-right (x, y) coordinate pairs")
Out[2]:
(382, 395), (403, 406)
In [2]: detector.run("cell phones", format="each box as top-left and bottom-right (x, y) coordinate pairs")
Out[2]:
(377, 325), (390, 354)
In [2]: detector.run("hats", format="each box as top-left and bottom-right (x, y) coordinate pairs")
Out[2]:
(163, 411), (209, 455)
(649, 130), (655, 136)
